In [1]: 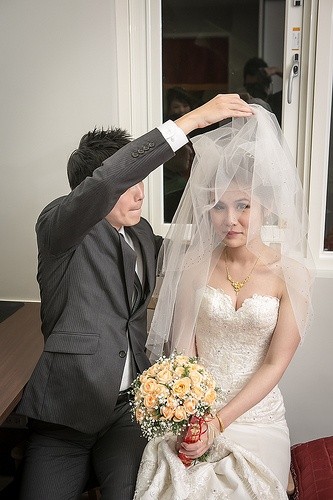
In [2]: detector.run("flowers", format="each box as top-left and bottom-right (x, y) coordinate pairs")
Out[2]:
(126, 349), (222, 466)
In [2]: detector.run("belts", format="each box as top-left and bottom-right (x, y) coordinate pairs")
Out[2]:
(115, 389), (135, 406)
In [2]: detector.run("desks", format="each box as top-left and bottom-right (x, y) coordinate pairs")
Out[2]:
(0, 302), (44, 428)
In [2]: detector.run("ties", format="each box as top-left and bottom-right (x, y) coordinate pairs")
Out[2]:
(120, 233), (142, 317)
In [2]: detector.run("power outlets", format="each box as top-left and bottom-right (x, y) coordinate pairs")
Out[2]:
(1, 412), (28, 428)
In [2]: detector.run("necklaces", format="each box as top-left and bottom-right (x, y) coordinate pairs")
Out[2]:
(223, 245), (263, 295)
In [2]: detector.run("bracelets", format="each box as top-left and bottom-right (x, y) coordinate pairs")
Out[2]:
(215, 413), (224, 434)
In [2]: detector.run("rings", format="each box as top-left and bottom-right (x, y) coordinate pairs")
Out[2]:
(199, 438), (204, 443)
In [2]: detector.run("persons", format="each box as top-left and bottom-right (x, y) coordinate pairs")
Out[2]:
(12, 93), (256, 499)
(241, 57), (287, 124)
(165, 86), (192, 117)
(133, 152), (314, 499)
(164, 143), (191, 223)
